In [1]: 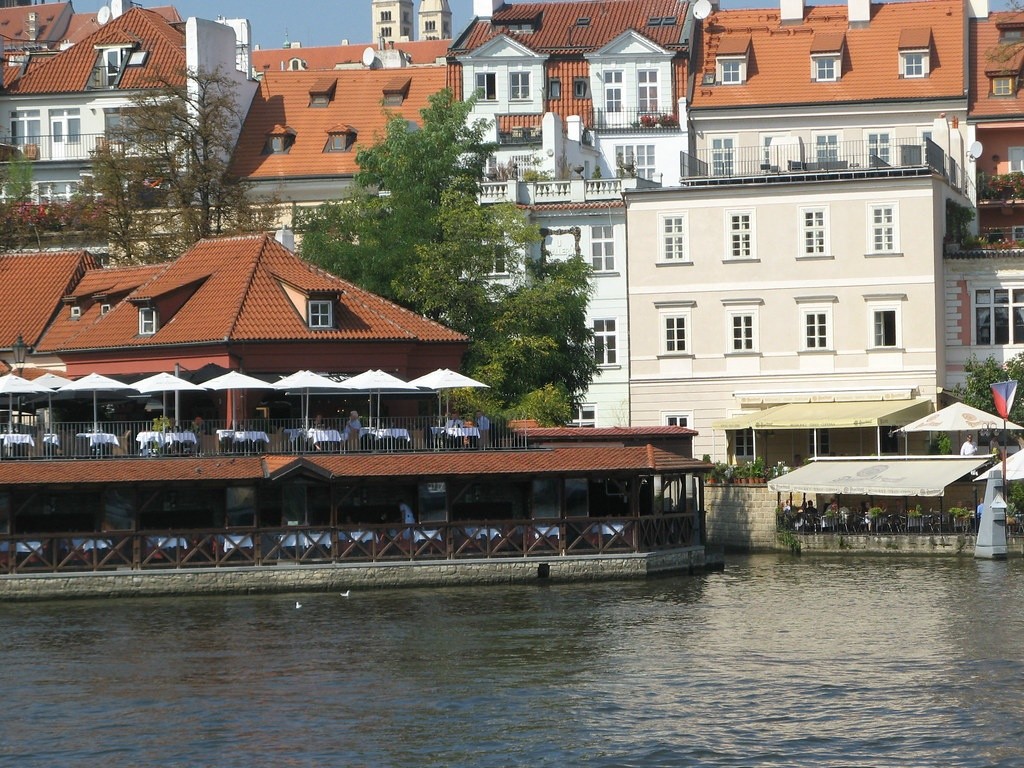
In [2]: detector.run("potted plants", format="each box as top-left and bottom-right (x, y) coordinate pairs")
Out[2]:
(703, 454), (769, 483)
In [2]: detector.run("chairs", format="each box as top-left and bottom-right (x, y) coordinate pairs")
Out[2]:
(792, 513), (971, 536)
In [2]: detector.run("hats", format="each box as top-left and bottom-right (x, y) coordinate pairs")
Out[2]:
(351, 411), (359, 418)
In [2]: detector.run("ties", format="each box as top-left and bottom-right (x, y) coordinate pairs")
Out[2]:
(452, 420), (455, 427)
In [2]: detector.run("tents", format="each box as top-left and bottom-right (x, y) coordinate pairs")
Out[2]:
(711, 393), (933, 466)
(767, 456), (995, 535)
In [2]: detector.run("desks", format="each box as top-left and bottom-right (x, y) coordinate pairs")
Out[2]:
(461, 526), (502, 541)
(42, 434), (59, 454)
(136, 427), (481, 452)
(146, 536), (188, 550)
(0, 541), (43, 557)
(279, 532), (331, 549)
(63, 539), (114, 550)
(76, 433), (120, 455)
(338, 532), (379, 545)
(587, 524), (627, 537)
(531, 526), (560, 541)
(0, 434), (35, 457)
(216, 534), (253, 554)
(392, 530), (442, 555)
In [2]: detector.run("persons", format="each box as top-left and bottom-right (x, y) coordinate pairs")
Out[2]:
(400, 506), (417, 542)
(989, 436), (1001, 455)
(784, 497), (987, 532)
(961, 435), (980, 457)
(314, 410), (490, 452)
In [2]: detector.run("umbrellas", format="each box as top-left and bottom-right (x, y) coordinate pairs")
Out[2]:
(972, 445), (1024, 481)
(893, 401), (1024, 443)
(0, 368), (490, 432)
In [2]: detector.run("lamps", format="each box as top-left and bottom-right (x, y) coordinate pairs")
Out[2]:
(573, 165), (587, 180)
(761, 163), (770, 174)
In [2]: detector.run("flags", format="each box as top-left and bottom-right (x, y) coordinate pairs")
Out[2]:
(989, 380), (1017, 421)
